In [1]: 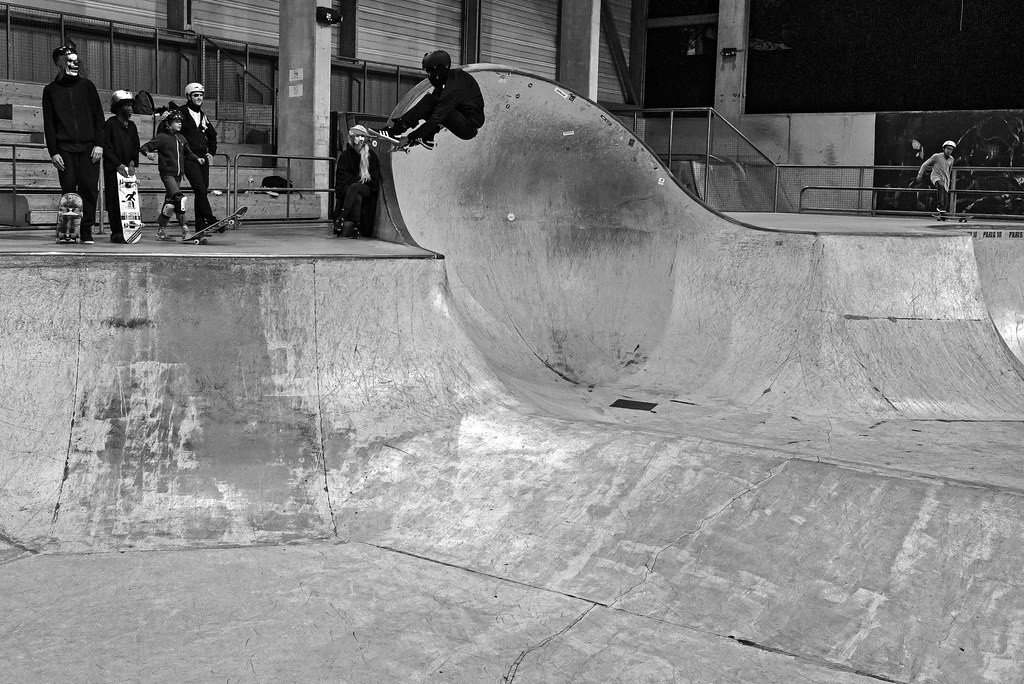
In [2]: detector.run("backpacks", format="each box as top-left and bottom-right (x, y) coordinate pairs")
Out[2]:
(132, 90), (155, 114)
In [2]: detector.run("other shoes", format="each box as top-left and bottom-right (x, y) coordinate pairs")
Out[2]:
(80, 227), (94, 244)
(111, 234), (128, 244)
(334, 218), (344, 230)
(351, 228), (360, 239)
(156, 231), (169, 238)
(937, 207), (946, 213)
(938, 217), (950, 221)
(182, 233), (192, 240)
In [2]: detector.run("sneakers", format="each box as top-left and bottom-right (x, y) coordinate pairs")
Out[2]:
(368, 126), (401, 144)
(407, 133), (435, 151)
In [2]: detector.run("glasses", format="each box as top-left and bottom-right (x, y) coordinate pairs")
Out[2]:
(192, 93), (204, 98)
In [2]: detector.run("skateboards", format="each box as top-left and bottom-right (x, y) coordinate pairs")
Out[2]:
(182, 206), (248, 245)
(931, 212), (973, 222)
(117, 166), (145, 244)
(56, 193), (83, 244)
(356, 134), (411, 154)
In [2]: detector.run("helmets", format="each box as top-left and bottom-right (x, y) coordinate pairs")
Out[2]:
(160, 109), (185, 128)
(111, 90), (134, 104)
(185, 82), (206, 99)
(422, 50), (452, 71)
(52, 44), (77, 66)
(942, 141), (956, 151)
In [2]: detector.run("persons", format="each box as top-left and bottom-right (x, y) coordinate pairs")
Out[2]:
(140, 110), (205, 241)
(903, 137), (932, 211)
(43, 44), (105, 245)
(102, 90), (140, 244)
(911, 141), (957, 221)
(157, 82), (229, 237)
(368, 50), (485, 150)
(333, 124), (380, 239)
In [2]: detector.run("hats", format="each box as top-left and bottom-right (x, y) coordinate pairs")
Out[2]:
(348, 125), (369, 137)
